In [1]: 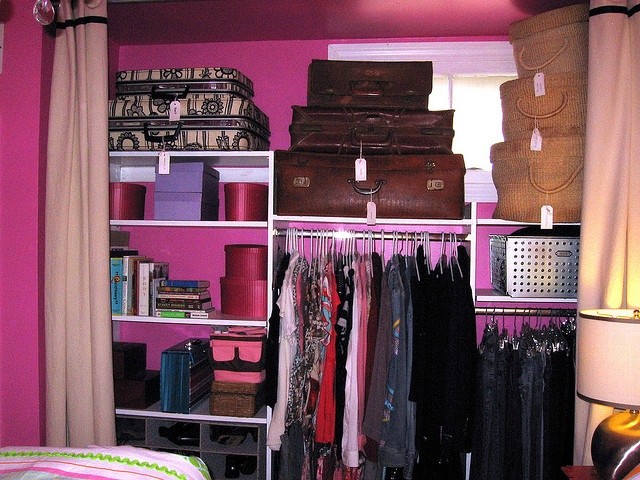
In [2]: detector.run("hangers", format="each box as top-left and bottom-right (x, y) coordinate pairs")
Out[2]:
(280, 228), (465, 288)
(484, 307), (576, 353)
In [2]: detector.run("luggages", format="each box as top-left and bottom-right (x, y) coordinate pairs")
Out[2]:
(116, 67), (255, 95)
(107, 95), (271, 151)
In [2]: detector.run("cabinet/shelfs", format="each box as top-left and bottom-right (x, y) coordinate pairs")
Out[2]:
(105, 151), (274, 480)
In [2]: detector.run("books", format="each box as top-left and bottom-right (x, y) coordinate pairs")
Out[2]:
(110, 231), (218, 319)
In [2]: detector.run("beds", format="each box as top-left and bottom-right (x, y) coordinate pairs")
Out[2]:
(0, 444), (212, 480)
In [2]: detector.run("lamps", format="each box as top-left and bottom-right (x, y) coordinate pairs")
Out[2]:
(574, 309), (640, 480)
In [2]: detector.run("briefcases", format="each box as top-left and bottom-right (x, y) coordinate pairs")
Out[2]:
(309, 58), (432, 107)
(275, 148), (463, 218)
(290, 102), (454, 152)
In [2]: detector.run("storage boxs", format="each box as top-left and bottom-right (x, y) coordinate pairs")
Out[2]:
(111, 342), (146, 383)
(224, 244), (267, 281)
(220, 276), (267, 318)
(209, 383), (266, 417)
(225, 183), (269, 220)
(109, 181), (147, 220)
(211, 325), (266, 382)
(152, 193), (218, 222)
(154, 163), (219, 198)
(114, 370), (161, 409)
(487, 235), (578, 298)
